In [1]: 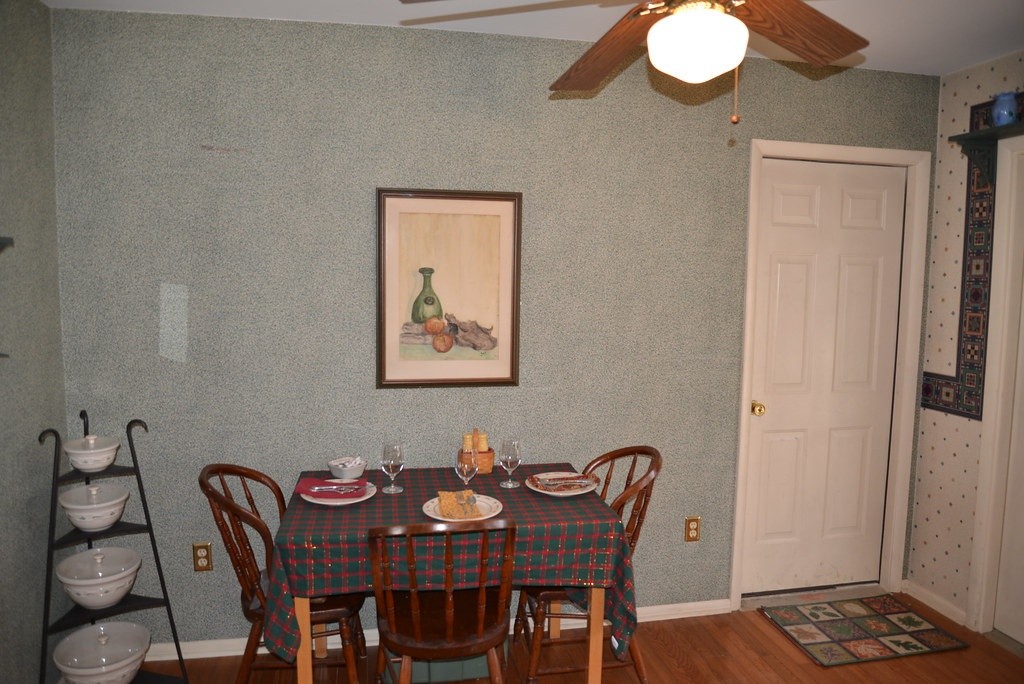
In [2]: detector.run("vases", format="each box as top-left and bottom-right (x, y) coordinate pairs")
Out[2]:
(989, 92), (1018, 127)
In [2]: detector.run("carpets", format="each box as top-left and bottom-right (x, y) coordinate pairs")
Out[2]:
(756, 592), (970, 668)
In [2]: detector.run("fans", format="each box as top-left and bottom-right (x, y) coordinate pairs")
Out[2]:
(403, 0), (871, 90)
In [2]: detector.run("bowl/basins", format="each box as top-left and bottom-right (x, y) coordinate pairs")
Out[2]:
(327, 456), (368, 478)
(58, 483), (130, 532)
(53, 621), (151, 684)
(62, 435), (120, 473)
(55, 547), (142, 609)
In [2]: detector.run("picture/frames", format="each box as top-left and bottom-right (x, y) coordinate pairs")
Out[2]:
(376, 188), (524, 386)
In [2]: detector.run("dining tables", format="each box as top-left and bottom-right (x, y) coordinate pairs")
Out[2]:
(261, 462), (637, 684)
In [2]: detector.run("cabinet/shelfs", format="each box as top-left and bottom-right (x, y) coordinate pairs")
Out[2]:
(38, 420), (190, 684)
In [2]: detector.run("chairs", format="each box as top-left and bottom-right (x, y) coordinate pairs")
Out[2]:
(515, 445), (661, 684)
(360, 518), (517, 684)
(198, 464), (367, 684)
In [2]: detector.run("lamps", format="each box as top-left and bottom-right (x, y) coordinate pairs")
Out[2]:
(648, 1), (750, 85)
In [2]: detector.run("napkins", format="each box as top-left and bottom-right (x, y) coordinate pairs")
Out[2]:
(293, 476), (365, 499)
(524, 472), (600, 491)
(435, 490), (484, 520)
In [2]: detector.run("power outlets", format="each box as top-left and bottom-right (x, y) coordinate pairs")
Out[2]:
(685, 518), (701, 541)
(193, 543), (213, 570)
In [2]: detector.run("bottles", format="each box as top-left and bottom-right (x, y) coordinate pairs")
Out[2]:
(477, 433), (488, 453)
(463, 433), (473, 454)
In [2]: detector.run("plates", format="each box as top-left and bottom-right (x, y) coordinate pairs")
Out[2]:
(525, 472), (597, 497)
(422, 494), (503, 522)
(301, 479), (376, 506)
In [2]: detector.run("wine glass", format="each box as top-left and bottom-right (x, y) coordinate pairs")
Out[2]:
(498, 439), (522, 488)
(380, 444), (406, 494)
(453, 448), (478, 493)
